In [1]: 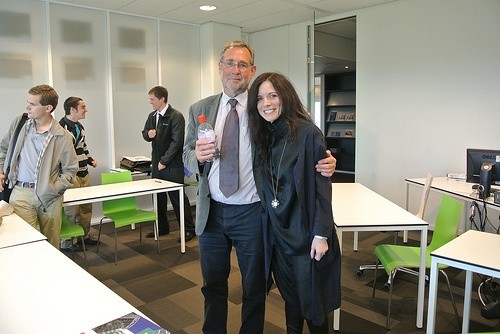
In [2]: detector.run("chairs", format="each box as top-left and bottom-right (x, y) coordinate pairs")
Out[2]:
(356, 175), (434, 293)
(372, 194), (463, 334)
(59, 206), (89, 267)
(96, 171), (161, 266)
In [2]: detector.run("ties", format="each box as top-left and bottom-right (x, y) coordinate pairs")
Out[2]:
(157, 113), (162, 129)
(219, 99), (239, 198)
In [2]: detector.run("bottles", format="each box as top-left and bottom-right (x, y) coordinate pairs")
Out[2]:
(197, 115), (220, 163)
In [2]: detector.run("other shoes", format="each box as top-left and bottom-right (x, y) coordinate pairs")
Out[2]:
(77, 235), (100, 245)
(146, 228), (169, 238)
(179, 231), (196, 243)
(61, 243), (86, 252)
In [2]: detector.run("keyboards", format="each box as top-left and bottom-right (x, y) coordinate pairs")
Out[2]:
(472, 185), (479, 189)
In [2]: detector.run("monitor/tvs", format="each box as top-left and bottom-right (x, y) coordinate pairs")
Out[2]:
(467, 149), (500, 200)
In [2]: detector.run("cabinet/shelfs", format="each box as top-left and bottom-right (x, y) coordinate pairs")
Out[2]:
(321, 72), (355, 181)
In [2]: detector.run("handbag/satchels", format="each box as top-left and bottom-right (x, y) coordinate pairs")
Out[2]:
(0, 183), (13, 203)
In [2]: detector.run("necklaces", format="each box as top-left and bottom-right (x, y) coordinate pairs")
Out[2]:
(270, 137), (287, 208)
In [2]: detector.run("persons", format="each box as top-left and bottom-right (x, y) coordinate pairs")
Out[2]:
(183, 41), (337, 334)
(246, 72), (342, 334)
(142, 86), (197, 243)
(0, 85), (79, 251)
(58, 97), (101, 252)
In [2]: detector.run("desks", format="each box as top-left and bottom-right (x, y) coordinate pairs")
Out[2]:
(0, 199), (48, 250)
(427, 230), (500, 334)
(325, 183), (430, 334)
(404, 176), (500, 244)
(0, 240), (174, 334)
(61, 178), (186, 253)
(110, 167), (152, 230)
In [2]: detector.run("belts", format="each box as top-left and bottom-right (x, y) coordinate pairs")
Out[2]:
(15, 181), (35, 188)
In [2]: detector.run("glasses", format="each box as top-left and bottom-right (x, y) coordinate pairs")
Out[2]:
(220, 60), (251, 71)
